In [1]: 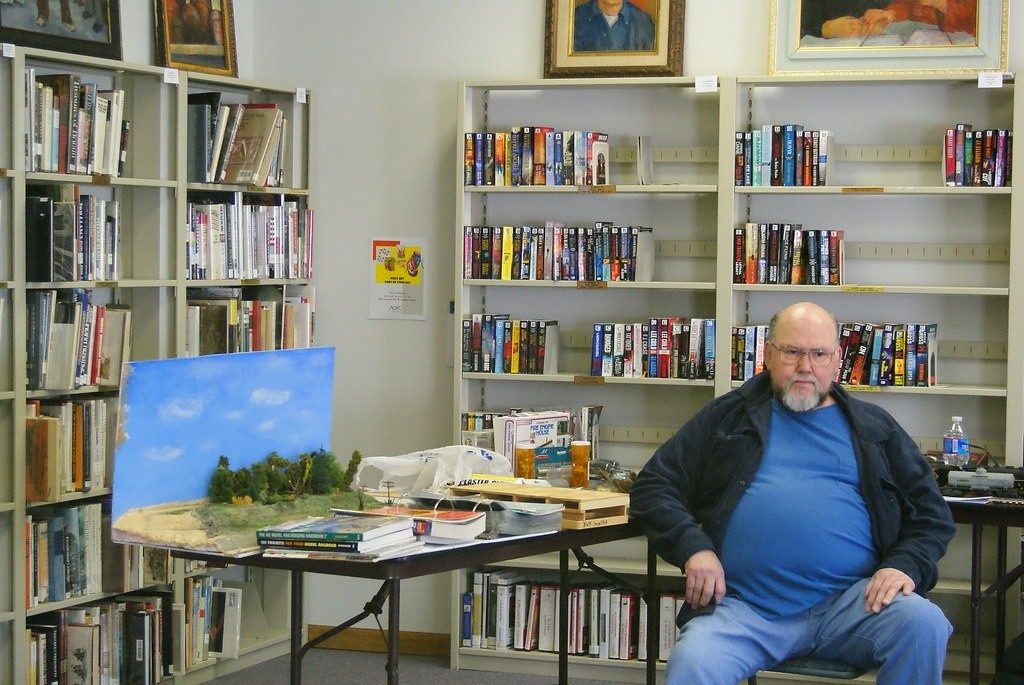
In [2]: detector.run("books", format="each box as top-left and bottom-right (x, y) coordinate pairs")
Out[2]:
(463, 123), (717, 383)
(733, 121), (1016, 387)
(0, 65), (318, 685)
(461, 571), (688, 666)
(459, 404), (603, 488)
(256, 490), (564, 563)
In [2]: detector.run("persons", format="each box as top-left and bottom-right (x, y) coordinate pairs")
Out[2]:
(627, 302), (957, 685)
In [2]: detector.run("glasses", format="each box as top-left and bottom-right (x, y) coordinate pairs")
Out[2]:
(769, 341), (836, 369)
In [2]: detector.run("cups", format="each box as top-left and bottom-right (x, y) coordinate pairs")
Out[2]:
(516, 444), (535, 479)
(570, 441), (591, 488)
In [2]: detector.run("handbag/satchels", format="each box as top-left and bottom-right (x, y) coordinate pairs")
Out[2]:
(349, 445), (512, 503)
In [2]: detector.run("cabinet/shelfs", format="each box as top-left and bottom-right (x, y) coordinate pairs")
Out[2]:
(946, 499), (1024, 684)
(450, 72), (1024, 683)
(0, 39), (315, 685)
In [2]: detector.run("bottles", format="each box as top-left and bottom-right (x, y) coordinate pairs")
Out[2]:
(942, 416), (970, 470)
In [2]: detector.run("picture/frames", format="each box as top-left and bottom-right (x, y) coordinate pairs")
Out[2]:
(0, 0), (122, 61)
(766, 0), (1010, 79)
(153, 0), (239, 78)
(543, 0), (685, 78)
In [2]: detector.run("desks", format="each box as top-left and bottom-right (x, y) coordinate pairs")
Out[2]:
(109, 487), (658, 684)
